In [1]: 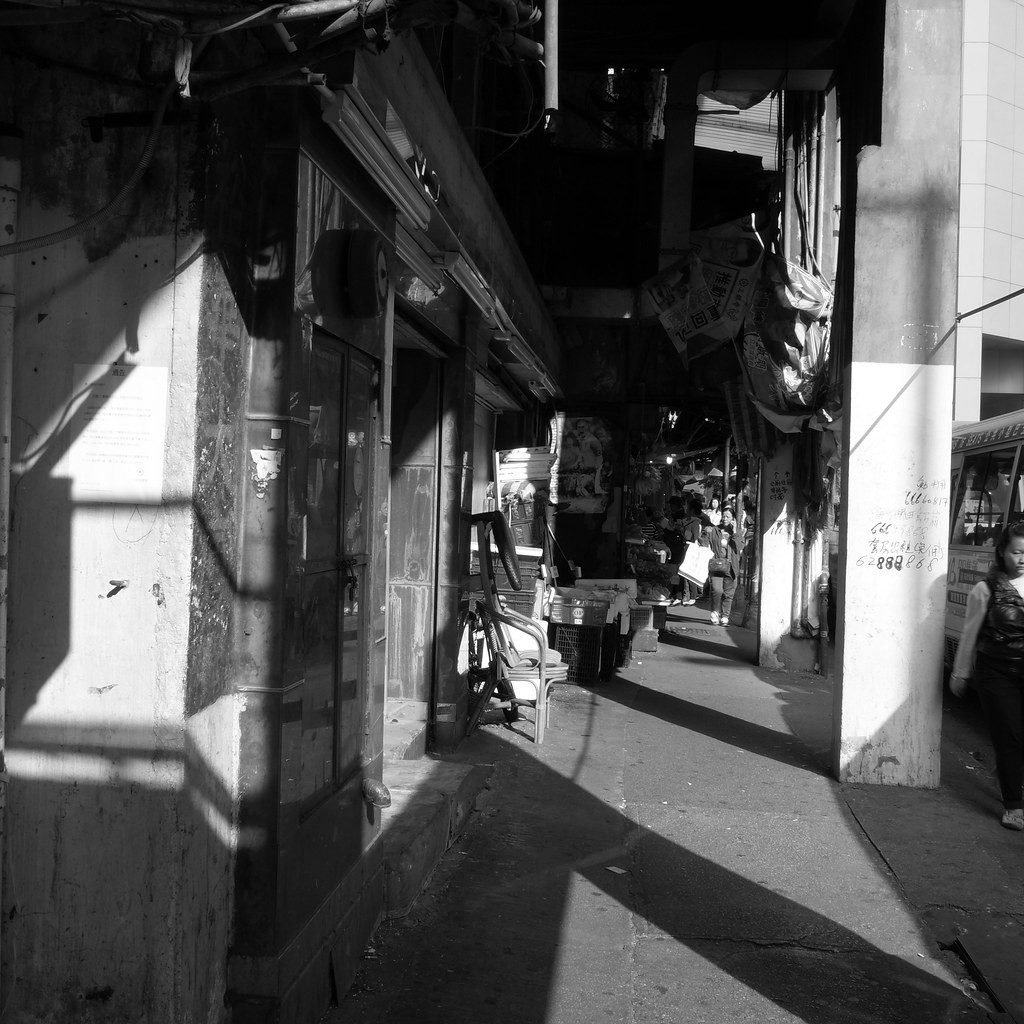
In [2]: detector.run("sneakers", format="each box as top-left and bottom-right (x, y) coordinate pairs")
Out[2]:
(711, 614), (720, 625)
(720, 617), (730, 627)
(1002, 809), (1024, 831)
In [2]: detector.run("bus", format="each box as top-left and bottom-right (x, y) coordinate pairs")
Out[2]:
(945, 409), (1024, 660)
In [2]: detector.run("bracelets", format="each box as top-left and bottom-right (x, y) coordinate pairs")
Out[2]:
(951, 673), (966, 681)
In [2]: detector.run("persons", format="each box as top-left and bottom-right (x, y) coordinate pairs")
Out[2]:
(695, 508), (745, 625)
(657, 491), (756, 605)
(950, 528), (1024, 831)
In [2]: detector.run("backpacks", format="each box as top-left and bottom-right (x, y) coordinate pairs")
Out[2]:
(667, 521), (695, 563)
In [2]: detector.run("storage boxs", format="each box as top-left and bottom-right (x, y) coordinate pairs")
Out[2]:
(549, 595), (663, 688)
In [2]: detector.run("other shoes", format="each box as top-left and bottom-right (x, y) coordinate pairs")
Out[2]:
(672, 598), (696, 606)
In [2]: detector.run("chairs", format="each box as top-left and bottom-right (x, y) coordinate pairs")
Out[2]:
(464, 591), (570, 748)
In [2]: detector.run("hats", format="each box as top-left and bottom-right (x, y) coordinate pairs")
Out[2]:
(666, 495), (682, 505)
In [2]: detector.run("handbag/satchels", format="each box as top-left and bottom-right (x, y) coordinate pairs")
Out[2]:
(678, 539), (715, 587)
(709, 558), (730, 578)
